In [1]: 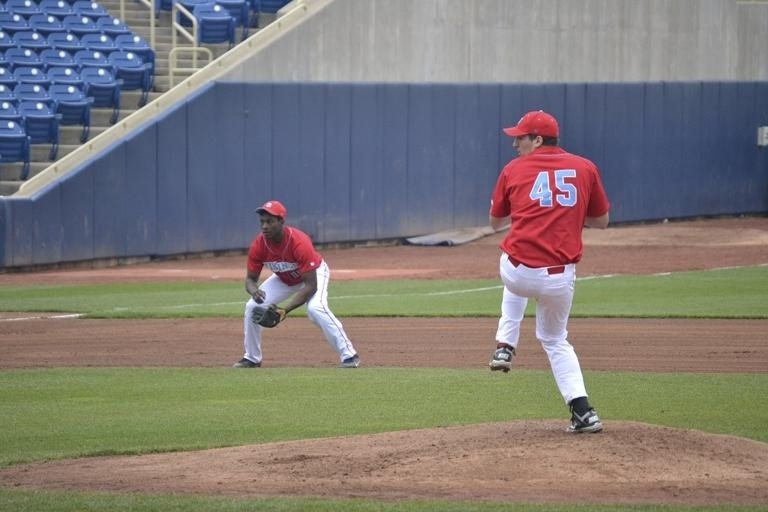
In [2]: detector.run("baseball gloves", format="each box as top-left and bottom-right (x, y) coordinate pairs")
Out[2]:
(252, 304), (285, 328)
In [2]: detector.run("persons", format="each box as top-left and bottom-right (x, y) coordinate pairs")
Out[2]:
(488, 108), (611, 433)
(229, 201), (362, 368)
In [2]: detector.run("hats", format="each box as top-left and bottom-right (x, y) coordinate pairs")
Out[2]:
(254, 200), (285, 221)
(502, 110), (558, 138)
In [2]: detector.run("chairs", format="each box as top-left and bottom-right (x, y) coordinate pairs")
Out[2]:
(0, 0), (158, 182)
(155, 1), (292, 60)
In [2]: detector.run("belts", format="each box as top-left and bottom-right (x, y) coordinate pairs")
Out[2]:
(508, 255), (564, 274)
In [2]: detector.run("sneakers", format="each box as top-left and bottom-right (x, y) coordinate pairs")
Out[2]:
(232, 358), (261, 368)
(338, 354), (360, 368)
(488, 348), (513, 372)
(566, 408), (602, 433)
